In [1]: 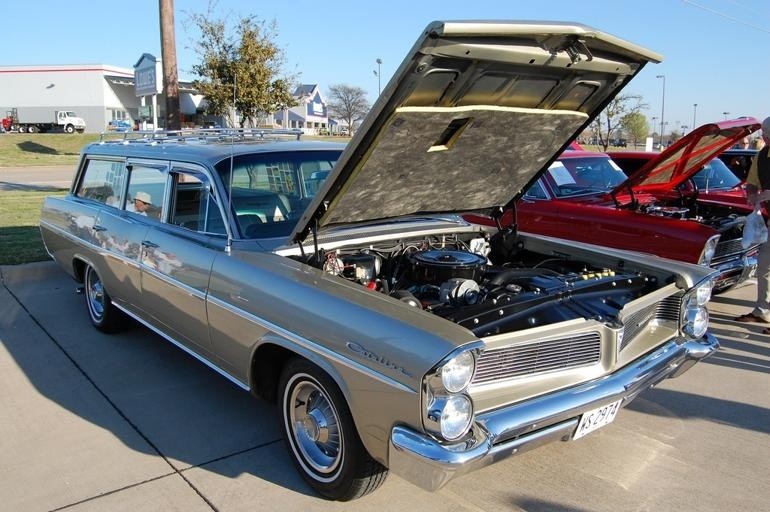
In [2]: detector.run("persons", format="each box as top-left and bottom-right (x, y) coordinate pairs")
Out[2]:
(730, 116), (770, 334)
(104, 191), (183, 276)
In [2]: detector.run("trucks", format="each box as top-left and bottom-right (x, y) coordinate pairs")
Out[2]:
(17, 110), (86, 134)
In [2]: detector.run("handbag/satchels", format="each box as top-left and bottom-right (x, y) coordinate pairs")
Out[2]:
(741, 206), (768, 249)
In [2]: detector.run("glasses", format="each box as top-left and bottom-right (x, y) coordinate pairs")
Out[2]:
(143, 202), (151, 205)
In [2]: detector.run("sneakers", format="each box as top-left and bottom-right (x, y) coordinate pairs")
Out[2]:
(733, 313), (770, 334)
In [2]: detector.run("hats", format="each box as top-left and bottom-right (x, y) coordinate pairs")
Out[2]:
(133, 192), (152, 205)
